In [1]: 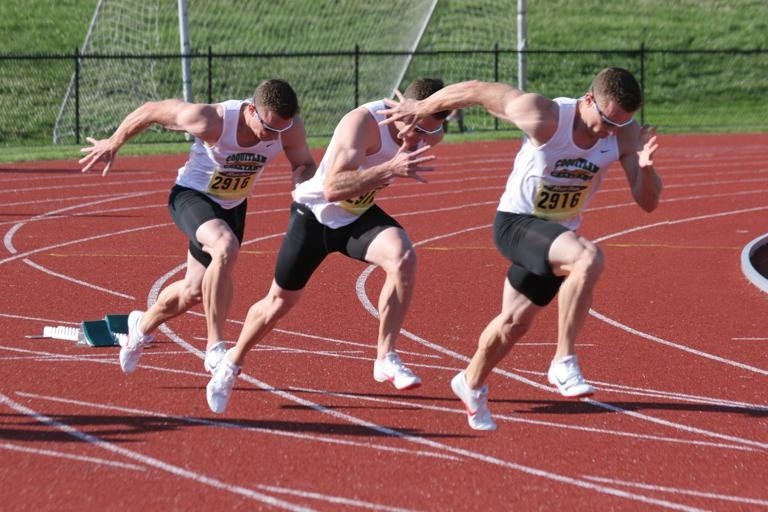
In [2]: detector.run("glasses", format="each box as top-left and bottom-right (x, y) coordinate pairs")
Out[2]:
(252, 97), (293, 135)
(413, 122), (442, 137)
(590, 86), (633, 129)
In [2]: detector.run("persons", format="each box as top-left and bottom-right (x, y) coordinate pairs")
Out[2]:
(203, 76), (453, 418)
(74, 77), (319, 377)
(372, 63), (666, 434)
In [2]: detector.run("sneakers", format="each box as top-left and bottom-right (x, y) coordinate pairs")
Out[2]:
(546, 355), (594, 398)
(119, 310), (151, 374)
(203, 341), (227, 375)
(373, 352), (421, 391)
(206, 347), (239, 415)
(450, 371), (496, 431)
